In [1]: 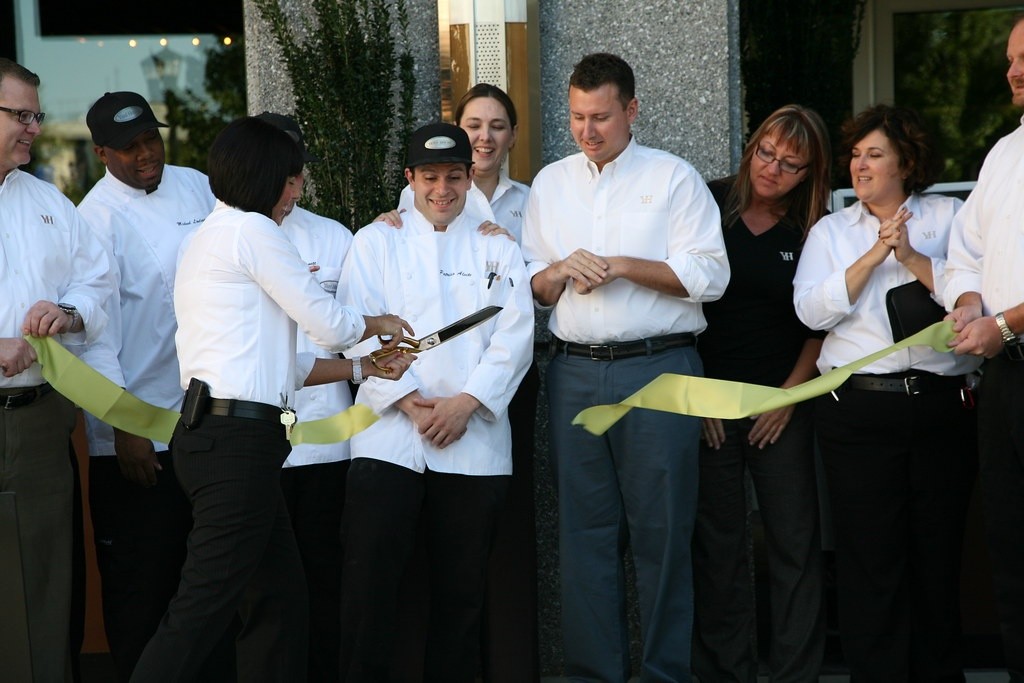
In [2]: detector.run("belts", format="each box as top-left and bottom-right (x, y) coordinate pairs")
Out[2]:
(1, 383), (55, 411)
(846, 375), (969, 397)
(554, 332), (696, 361)
(206, 399), (282, 423)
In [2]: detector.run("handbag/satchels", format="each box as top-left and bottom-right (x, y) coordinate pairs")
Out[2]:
(886, 277), (948, 343)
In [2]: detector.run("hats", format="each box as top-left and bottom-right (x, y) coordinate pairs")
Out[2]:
(406, 123), (476, 167)
(256, 111), (322, 162)
(86, 91), (170, 148)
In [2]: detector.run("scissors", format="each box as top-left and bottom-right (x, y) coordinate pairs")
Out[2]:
(370, 305), (504, 371)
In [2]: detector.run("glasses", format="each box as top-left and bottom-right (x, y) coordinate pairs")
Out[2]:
(755, 142), (815, 175)
(0, 106), (46, 126)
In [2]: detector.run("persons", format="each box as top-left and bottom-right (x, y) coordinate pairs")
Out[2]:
(0, 14), (1024, 682)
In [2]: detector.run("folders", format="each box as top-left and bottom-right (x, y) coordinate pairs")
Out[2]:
(886, 281), (950, 343)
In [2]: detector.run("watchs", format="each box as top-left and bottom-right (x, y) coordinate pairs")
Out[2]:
(350, 356), (367, 385)
(995, 313), (1020, 346)
(57, 302), (79, 332)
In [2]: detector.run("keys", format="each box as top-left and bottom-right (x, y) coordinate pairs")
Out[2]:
(281, 410), (296, 440)
